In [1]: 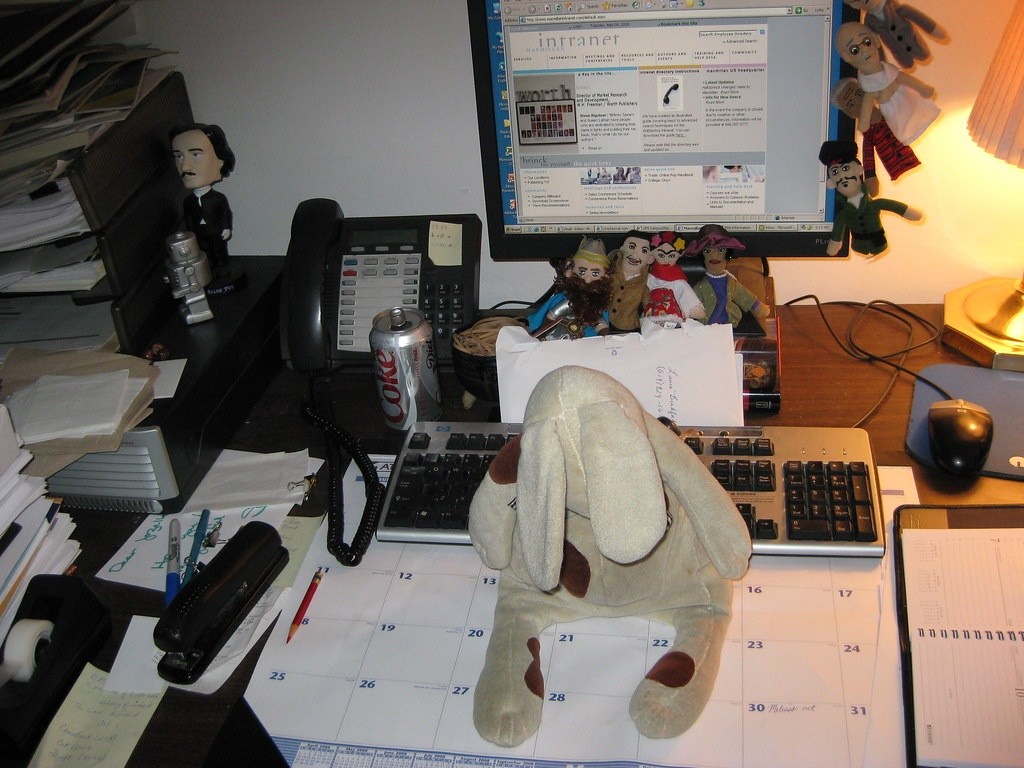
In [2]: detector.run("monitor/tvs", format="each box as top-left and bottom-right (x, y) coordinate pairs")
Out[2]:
(467, 0), (861, 338)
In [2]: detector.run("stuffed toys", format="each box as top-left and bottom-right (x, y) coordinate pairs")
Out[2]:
(467, 361), (756, 747)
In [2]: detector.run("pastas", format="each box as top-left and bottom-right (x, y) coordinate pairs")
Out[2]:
(451, 316), (530, 357)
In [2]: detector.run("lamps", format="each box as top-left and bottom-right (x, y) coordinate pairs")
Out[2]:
(941, 0), (1024, 373)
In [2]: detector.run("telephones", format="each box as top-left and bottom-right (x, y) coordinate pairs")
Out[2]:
(278, 197), (482, 375)
(664, 84), (679, 103)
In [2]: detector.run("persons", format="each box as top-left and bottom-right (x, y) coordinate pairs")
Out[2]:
(703, 164), (764, 183)
(169, 121), (235, 281)
(587, 167), (641, 183)
(684, 223), (772, 328)
(831, 78), (921, 197)
(844, 0), (946, 72)
(836, 21), (941, 146)
(602, 230), (654, 335)
(527, 234), (613, 342)
(639, 231), (707, 329)
(817, 139), (921, 260)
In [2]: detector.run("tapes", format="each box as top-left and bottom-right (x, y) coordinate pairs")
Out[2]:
(4, 618), (54, 684)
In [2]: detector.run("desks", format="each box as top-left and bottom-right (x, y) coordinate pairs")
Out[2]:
(71, 303), (1024, 768)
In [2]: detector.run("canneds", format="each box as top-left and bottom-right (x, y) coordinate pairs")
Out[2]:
(367, 305), (444, 431)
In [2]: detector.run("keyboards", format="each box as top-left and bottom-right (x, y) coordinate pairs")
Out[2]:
(376, 420), (887, 559)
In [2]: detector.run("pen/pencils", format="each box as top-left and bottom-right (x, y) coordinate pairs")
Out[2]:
(286, 571), (323, 644)
(165, 509), (210, 607)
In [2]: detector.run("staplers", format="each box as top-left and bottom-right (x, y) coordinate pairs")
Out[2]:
(153, 520), (290, 685)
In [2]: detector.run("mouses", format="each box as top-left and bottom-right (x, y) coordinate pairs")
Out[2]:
(928, 399), (994, 474)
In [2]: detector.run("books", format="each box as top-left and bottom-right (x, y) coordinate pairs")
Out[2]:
(0, 0), (182, 294)
(0, 404), (83, 656)
